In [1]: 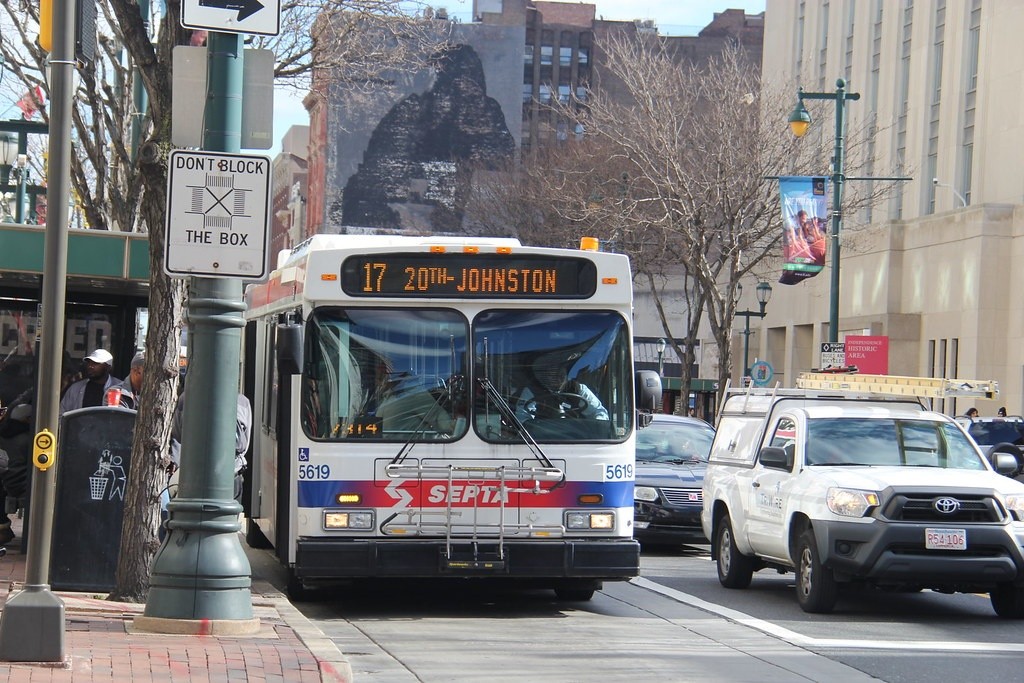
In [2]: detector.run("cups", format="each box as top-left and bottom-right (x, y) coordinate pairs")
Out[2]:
(106, 388), (120, 406)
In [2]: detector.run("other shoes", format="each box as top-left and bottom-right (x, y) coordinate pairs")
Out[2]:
(0, 546), (6, 557)
(0, 521), (15, 545)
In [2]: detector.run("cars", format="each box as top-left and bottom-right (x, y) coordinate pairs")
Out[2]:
(610, 414), (716, 545)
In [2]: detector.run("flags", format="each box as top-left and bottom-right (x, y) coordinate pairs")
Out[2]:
(15, 86), (44, 119)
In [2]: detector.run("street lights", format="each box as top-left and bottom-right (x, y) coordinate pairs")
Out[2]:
(788, 77), (861, 343)
(728, 278), (773, 393)
(657, 336), (666, 377)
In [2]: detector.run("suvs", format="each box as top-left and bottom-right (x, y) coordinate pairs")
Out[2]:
(699, 394), (1024, 619)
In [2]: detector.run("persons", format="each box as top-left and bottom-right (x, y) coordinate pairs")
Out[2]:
(966, 408), (978, 417)
(783, 209), (826, 246)
(0, 349), (251, 556)
(516, 360), (610, 427)
(688, 408), (695, 417)
(998, 407), (1006, 417)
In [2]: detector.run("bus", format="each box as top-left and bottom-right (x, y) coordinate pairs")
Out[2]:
(243, 234), (663, 603)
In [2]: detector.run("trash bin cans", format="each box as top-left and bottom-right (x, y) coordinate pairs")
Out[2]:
(48, 406), (138, 593)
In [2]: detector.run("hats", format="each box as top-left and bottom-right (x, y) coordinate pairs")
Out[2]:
(83, 349), (113, 368)
